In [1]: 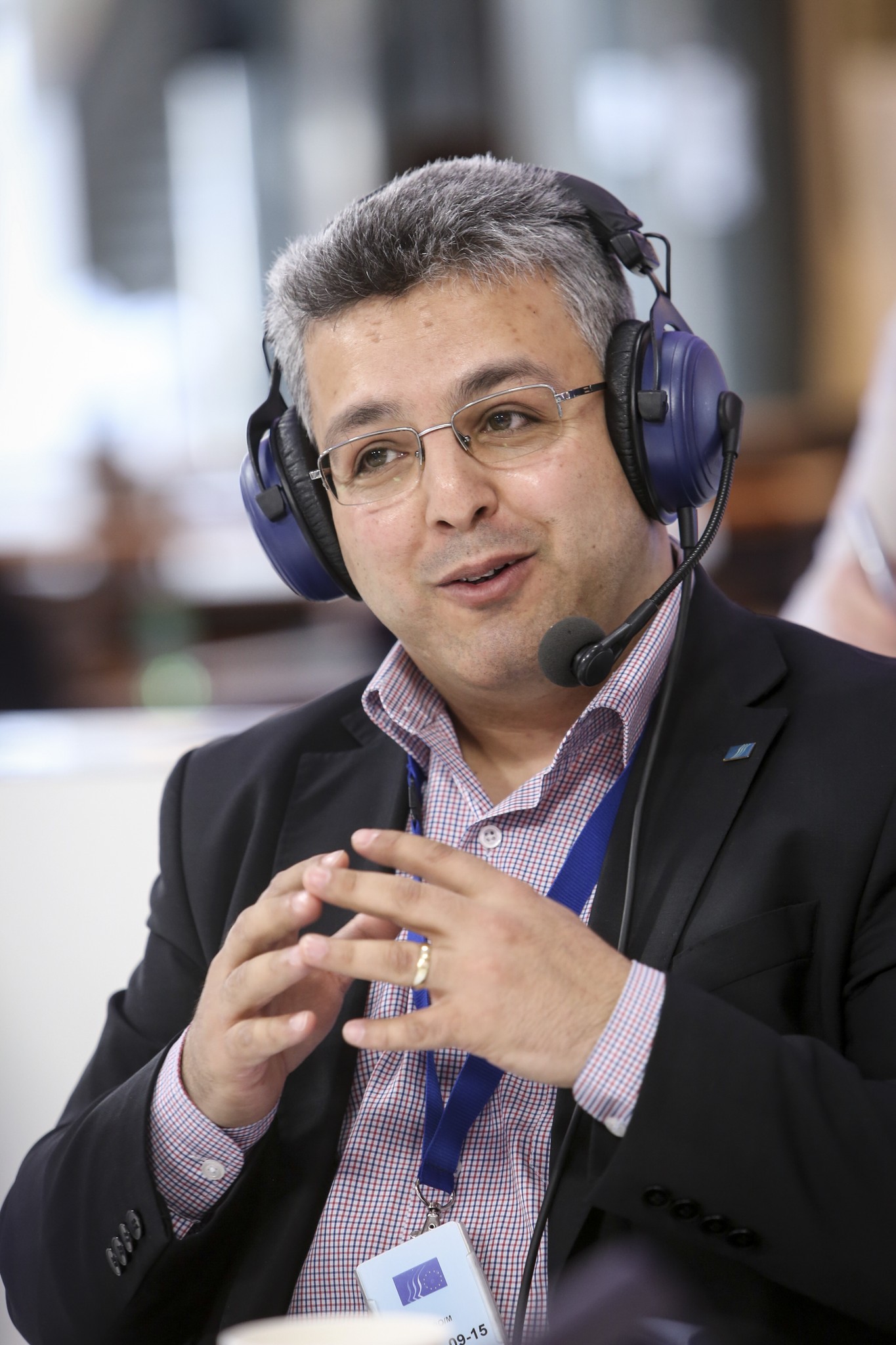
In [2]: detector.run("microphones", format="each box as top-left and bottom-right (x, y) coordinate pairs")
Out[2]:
(537, 392), (742, 689)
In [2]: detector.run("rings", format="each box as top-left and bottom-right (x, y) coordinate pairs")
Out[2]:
(411, 944), (431, 994)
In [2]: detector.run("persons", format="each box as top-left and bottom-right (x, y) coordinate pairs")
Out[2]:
(0, 148), (896, 1345)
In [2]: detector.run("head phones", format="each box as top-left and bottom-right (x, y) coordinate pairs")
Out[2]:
(231, 165), (744, 598)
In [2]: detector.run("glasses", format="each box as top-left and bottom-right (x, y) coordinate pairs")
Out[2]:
(310, 380), (605, 506)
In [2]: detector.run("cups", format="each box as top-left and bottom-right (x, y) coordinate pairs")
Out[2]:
(215, 1312), (452, 1345)
